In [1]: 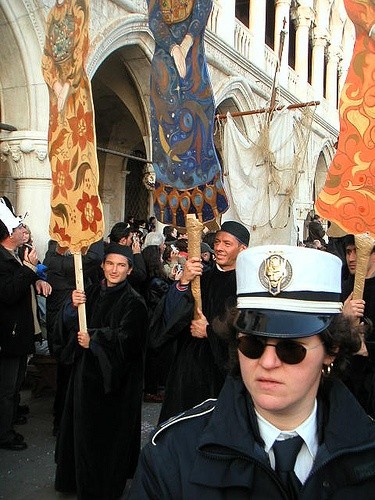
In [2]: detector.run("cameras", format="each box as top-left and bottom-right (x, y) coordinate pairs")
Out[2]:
(22, 243), (32, 254)
(132, 235), (139, 243)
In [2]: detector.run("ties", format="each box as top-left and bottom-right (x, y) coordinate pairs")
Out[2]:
(270, 436), (307, 500)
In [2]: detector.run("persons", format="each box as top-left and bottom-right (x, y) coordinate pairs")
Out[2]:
(0, 203), (52, 452)
(43, 216), (215, 435)
(331, 234), (375, 417)
(127, 245), (375, 500)
(300, 215), (327, 250)
(13, 225), (45, 425)
(148, 221), (250, 427)
(51, 244), (148, 500)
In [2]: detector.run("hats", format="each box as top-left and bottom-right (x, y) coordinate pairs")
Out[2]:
(219, 221), (250, 246)
(201, 242), (216, 254)
(104, 244), (133, 264)
(233, 245), (343, 338)
(0, 196), (22, 243)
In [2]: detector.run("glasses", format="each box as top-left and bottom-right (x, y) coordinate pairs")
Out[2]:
(123, 223), (131, 234)
(234, 336), (326, 365)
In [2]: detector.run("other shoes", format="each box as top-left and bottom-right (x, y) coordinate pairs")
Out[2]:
(145, 392), (165, 404)
(14, 434), (24, 441)
(13, 414), (28, 424)
(1, 439), (27, 450)
(17, 404), (31, 414)
